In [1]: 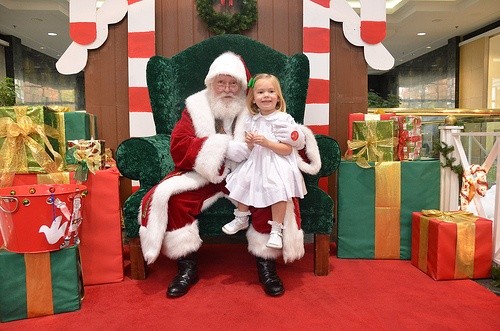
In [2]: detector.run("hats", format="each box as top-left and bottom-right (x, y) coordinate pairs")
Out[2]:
(204, 51), (252, 98)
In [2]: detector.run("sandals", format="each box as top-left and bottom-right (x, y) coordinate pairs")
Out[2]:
(266, 230), (283, 249)
(222, 217), (252, 236)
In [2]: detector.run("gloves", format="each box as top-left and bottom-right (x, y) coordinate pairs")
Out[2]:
(274, 123), (305, 150)
(226, 141), (251, 162)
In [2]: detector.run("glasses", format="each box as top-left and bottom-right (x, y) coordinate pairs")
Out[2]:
(215, 82), (239, 92)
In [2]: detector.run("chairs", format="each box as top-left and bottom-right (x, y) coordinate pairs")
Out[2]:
(116, 37), (342, 281)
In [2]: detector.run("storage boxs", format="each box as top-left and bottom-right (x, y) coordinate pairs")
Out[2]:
(0, 244), (80, 322)
(335, 113), (493, 282)
(0, 105), (109, 173)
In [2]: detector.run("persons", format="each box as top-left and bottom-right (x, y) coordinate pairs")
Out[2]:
(138, 50), (323, 297)
(221, 72), (305, 251)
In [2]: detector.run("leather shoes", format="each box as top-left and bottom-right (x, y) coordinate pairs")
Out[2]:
(165, 259), (199, 299)
(257, 259), (288, 296)
